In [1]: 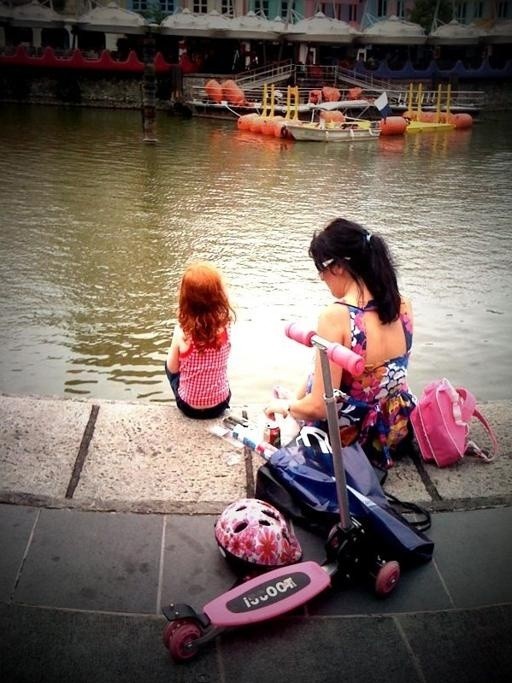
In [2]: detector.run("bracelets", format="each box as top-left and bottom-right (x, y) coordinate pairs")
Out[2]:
(282, 399), (289, 419)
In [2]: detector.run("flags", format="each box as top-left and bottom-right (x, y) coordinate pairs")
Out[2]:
(373, 91), (392, 118)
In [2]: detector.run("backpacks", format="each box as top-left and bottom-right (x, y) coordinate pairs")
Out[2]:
(410, 376), (498, 469)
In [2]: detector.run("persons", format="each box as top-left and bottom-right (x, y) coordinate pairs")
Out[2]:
(263, 215), (414, 469)
(165, 263), (232, 421)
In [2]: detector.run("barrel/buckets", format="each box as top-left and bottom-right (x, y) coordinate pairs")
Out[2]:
(381, 110), (473, 136)
(237, 112), (301, 137)
(204, 79), (224, 102)
(222, 79), (247, 106)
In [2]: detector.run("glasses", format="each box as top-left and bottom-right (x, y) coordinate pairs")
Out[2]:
(314, 256), (351, 271)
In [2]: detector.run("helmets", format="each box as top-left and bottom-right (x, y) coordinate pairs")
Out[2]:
(213, 496), (304, 568)
